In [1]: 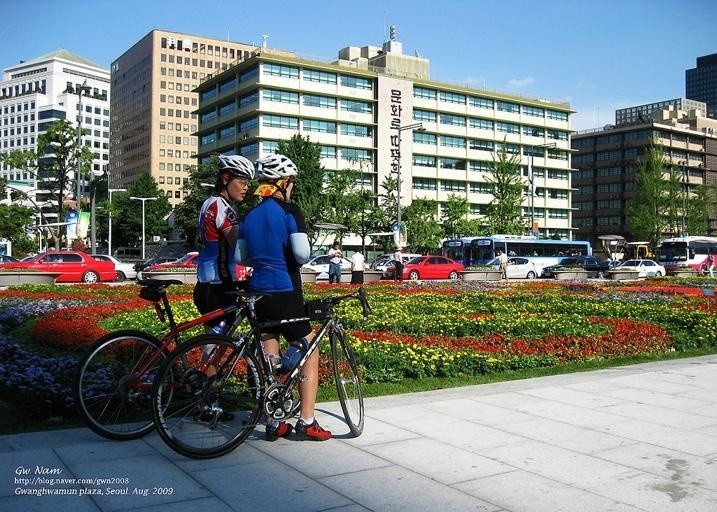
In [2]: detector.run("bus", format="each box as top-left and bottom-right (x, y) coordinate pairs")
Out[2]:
(655, 234), (717, 279)
(619, 240), (657, 263)
(440, 233), (538, 270)
(468, 238), (594, 279)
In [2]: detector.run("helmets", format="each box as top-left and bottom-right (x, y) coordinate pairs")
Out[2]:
(216, 153), (256, 181)
(253, 152), (299, 180)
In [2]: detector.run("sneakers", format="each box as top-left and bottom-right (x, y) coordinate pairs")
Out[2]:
(295, 417), (331, 442)
(265, 420), (293, 442)
(193, 404), (235, 423)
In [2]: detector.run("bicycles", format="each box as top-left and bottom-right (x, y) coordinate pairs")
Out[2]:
(67, 273), (326, 449)
(144, 286), (393, 461)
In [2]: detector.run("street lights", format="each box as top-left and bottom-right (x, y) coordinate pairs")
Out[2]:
(677, 159), (707, 238)
(528, 141), (562, 234)
(197, 180), (216, 191)
(395, 120), (430, 247)
(128, 193), (161, 260)
(104, 186), (129, 257)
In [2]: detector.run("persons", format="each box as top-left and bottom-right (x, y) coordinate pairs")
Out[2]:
(394, 248), (403, 281)
(498, 250), (507, 280)
(557, 248), (579, 257)
(192, 154), (255, 422)
(508, 249), (517, 256)
(351, 246), (365, 283)
(328, 241), (342, 284)
(708, 253), (715, 277)
(240, 153), (333, 441)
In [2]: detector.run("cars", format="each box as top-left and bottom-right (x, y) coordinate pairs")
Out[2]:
(294, 243), (466, 287)
(605, 258), (669, 282)
(0, 243), (256, 289)
(600, 257), (623, 279)
(482, 255), (542, 280)
(543, 250), (610, 282)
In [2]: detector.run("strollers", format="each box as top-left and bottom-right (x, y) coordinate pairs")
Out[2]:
(697, 262), (711, 279)
